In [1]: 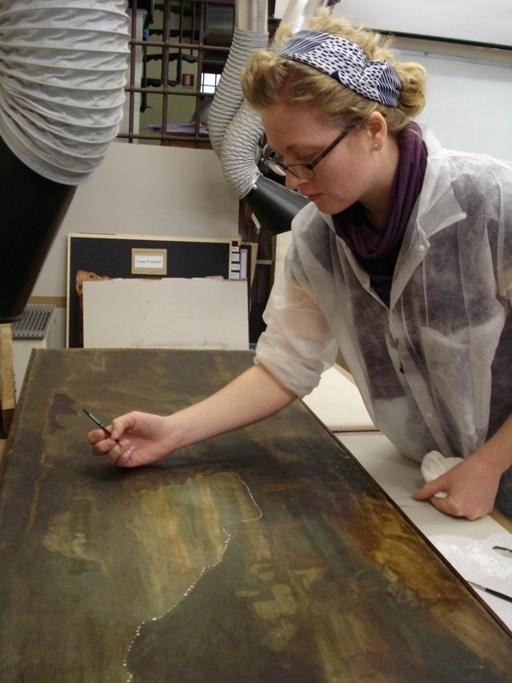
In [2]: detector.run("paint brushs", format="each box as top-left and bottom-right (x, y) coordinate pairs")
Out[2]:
(467, 580), (512, 604)
(81, 407), (131, 456)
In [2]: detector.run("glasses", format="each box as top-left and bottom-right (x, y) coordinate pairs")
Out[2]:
(264, 116), (361, 179)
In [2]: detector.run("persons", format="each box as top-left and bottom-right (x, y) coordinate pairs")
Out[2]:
(87, 17), (512, 523)
